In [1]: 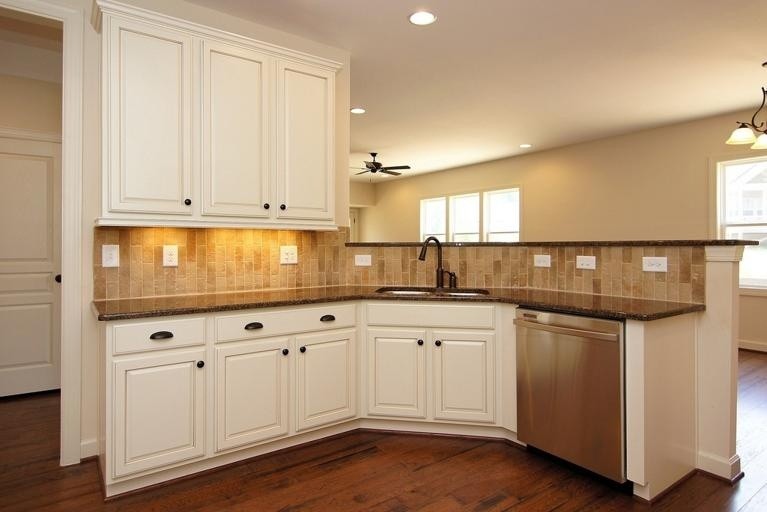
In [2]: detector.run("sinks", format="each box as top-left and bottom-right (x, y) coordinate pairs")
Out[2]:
(375, 286), (437, 296)
(438, 288), (489, 296)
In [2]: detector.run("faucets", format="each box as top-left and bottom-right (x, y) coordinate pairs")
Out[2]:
(419, 236), (444, 288)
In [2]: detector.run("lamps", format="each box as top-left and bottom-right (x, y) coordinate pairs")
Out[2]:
(726, 62), (767, 150)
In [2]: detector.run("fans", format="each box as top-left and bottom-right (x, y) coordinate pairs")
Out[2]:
(351, 152), (410, 176)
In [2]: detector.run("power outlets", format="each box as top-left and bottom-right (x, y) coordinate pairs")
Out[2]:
(163, 245), (179, 266)
(642, 256), (668, 273)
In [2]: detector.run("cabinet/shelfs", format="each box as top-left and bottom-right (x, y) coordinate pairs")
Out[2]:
(99, 314), (210, 500)
(210, 305), (362, 470)
(90, 1), (197, 229)
(362, 304), (501, 439)
(201, 38), (340, 233)
